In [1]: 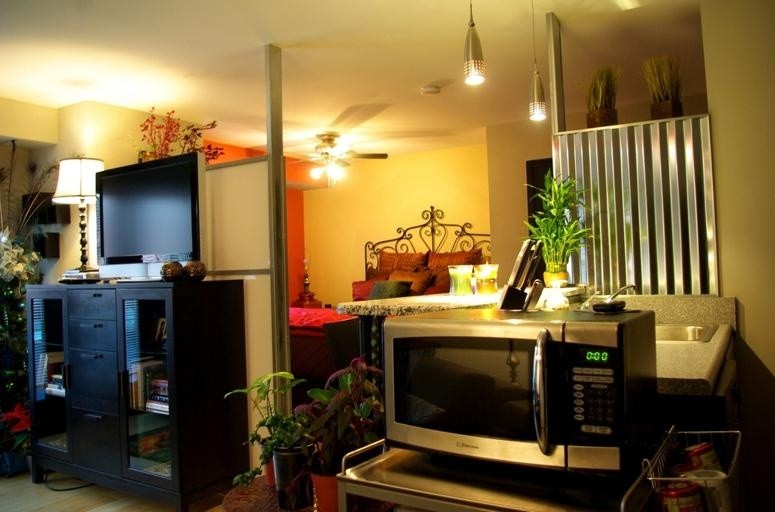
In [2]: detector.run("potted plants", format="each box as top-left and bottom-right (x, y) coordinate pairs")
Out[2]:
(520, 170), (596, 286)
(224, 357), (384, 512)
(586, 65), (618, 128)
(643, 54), (684, 120)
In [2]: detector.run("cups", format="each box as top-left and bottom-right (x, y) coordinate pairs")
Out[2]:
(473, 263), (502, 294)
(448, 262), (473, 297)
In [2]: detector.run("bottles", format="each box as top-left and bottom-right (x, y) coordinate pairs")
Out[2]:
(688, 325), (703, 341)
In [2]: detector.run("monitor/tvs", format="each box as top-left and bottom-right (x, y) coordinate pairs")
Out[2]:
(96, 151), (200, 283)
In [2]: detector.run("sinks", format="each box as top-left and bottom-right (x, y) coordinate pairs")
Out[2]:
(654, 325), (718, 342)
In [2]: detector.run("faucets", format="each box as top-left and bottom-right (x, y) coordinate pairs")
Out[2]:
(610, 285), (636, 299)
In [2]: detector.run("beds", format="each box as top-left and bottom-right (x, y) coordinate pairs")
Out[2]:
(289, 206), (492, 379)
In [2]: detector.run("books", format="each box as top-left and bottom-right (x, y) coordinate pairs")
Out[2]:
(128, 355), (170, 417)
(35, 350), (65, 397)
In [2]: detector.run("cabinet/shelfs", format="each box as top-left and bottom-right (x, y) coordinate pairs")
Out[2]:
(25, 280), (250, 512)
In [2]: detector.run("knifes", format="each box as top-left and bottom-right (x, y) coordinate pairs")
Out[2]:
(508, 239), (544, 292)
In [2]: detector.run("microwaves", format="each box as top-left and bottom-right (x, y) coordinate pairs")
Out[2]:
(380, 307), (658, 469)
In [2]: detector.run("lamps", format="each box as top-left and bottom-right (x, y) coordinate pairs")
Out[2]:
(529, 0), (547, 120)
(464, 0), (485, 86)
(51, 158), (104, 284)
(291, 247), (322, 308)
(310, 152), (347, 181)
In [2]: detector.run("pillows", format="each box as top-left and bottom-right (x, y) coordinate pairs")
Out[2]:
(353, 247), (483, 302)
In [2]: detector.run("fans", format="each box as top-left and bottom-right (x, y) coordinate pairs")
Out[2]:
(285, 134), (387, 169)
